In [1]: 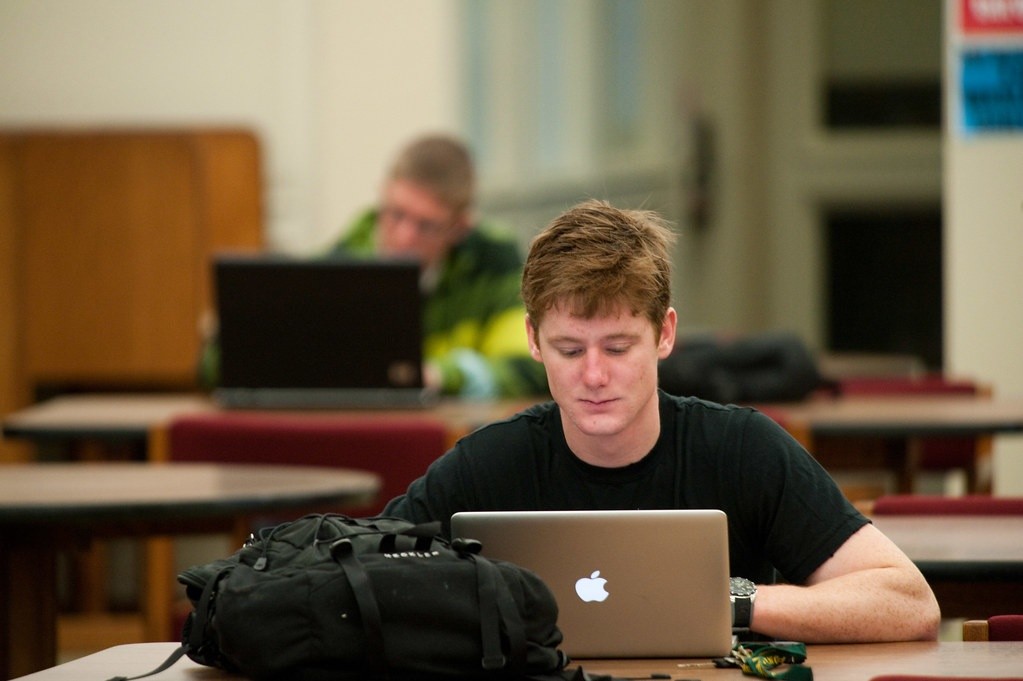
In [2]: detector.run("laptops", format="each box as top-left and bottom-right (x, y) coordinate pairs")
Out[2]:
(214, 258), (432, 409)
(450, 510), (731, 658)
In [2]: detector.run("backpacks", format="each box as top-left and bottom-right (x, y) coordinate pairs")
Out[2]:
(656, 327), (844, 409)
(178, 513), (568, 681)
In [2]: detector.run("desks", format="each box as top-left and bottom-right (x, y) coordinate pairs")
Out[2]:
(0, 387), (1023, 681)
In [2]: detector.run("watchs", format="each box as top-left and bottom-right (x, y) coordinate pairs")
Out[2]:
(729, 576), (756, 635)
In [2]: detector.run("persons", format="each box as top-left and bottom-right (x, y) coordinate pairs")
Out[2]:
(375, 200), (940, 641)
(200, 132), (546, 397)
